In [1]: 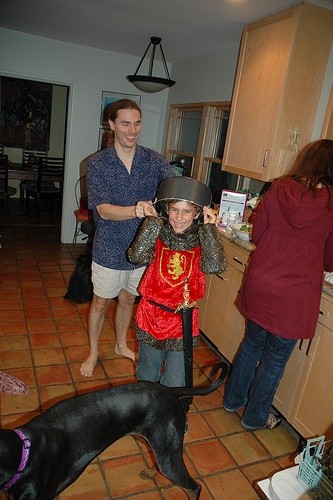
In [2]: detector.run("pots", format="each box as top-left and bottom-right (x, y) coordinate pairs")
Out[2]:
(150, 176), (214, 224)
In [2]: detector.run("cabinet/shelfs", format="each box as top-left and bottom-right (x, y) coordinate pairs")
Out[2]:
(221, 2), (333, 183)
(197, 225), (333, 444)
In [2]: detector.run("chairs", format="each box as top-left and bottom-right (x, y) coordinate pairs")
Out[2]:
(0, 155), (9, 218)
(19, 148), (54, 210)
(72, 176), (94, 253)
(0, 144), (16, 206)
(25, 156), (65, 226)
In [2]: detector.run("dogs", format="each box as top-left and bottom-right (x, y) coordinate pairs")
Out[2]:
(0, 362), (229, 500)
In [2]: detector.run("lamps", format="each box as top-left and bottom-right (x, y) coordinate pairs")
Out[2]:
(126, 36), (176, 93)
(4, 86), (49, 131)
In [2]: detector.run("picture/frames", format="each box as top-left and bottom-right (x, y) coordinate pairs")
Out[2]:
(100, 90), (141, 125)
(97, 128), (114, 151)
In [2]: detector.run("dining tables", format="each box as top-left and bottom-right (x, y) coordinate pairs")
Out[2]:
(0, 163), (64, 182)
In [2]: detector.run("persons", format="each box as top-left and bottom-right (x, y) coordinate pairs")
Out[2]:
(221, 139), (333, 431)
(80, 98), (177, 378)
(128, 200), (227, 434)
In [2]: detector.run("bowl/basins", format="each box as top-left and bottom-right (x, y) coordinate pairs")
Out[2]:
(230, 224), (254, 242)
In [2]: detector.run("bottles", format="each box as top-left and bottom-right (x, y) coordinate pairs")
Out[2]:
(226, 214), (236, 234)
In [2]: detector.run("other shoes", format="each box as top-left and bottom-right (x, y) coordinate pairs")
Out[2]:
(242, 413), (283, 429)
